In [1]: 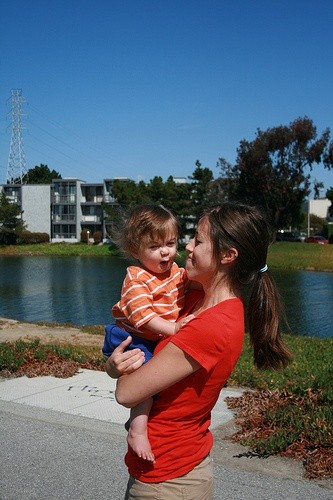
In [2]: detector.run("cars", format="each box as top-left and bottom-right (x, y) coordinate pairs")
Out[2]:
(281, 232), (305, 242)
(304, 237), (328, 244)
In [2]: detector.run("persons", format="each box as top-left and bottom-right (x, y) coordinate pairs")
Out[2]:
(105, 200), (295, 500)
(102, 203), (203, 464)
